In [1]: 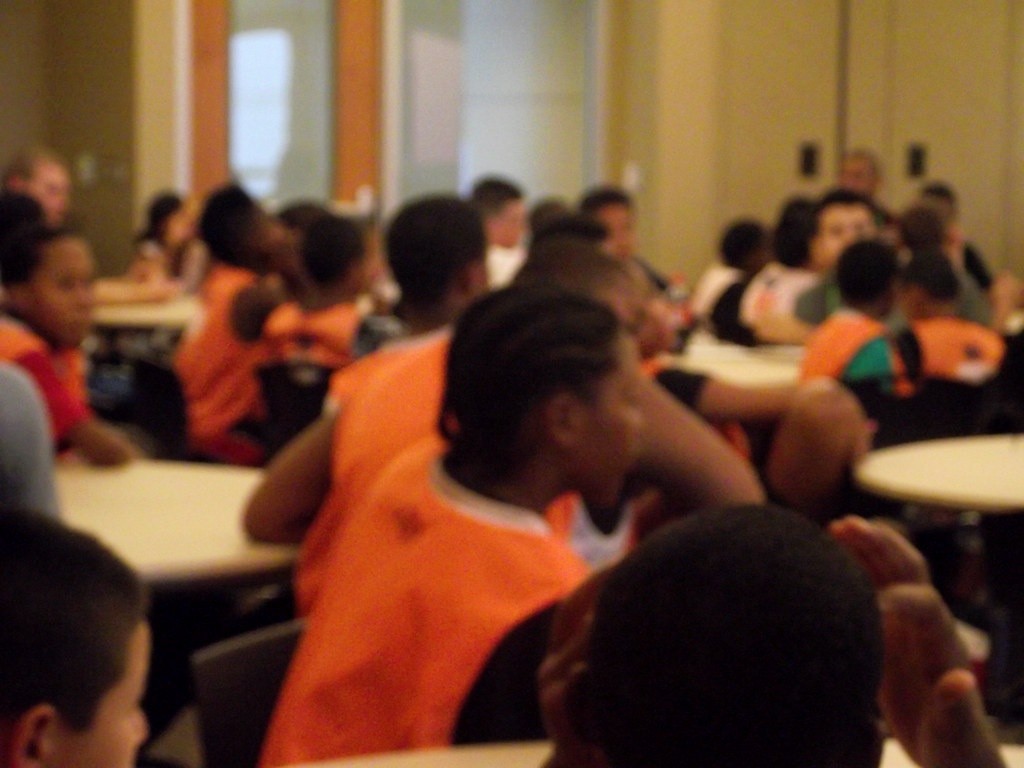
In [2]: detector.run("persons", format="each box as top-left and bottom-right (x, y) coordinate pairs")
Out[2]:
(1, 140), (1024, 768)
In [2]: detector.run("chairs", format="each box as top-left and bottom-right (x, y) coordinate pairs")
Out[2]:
(188, 610), (545, 768)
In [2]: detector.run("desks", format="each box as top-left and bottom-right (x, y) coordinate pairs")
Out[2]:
(89, 298), (193, 327)
(54, 454), (300, 588)
(854, 432), (1023, 515)
